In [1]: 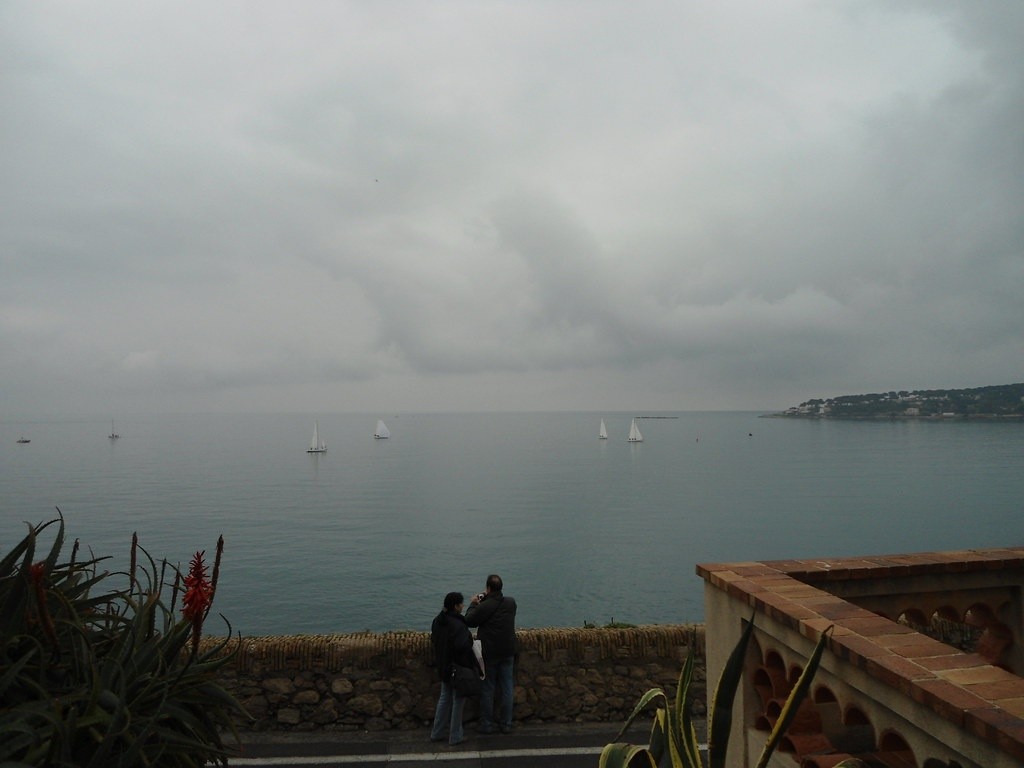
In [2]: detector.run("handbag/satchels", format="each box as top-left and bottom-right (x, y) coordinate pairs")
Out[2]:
(472, 640), (486, 680)
(447, 664), (479, 698)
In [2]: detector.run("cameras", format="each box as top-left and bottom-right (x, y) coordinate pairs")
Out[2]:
(476, 593), (486, 600)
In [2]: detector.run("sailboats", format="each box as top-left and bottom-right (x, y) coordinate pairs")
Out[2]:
(598, 418), (608, 439)
(374, 420), (391, 439)
(108, 420), (119, 438)
(307, 420), (328, 452)
(628, 417), (642, 442)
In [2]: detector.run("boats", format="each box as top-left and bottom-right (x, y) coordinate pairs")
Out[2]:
(17, 437), (31, 443)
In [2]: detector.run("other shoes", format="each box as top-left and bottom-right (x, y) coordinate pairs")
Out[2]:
(476, 725), (492, 734)
(431, 736), (448, 742)
(501, 726), (511, 733)
(449, 737), (468, 745)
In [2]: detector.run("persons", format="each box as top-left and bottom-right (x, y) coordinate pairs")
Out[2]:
(464, 575), (517, 734)
(430, 592), (477, 745)
